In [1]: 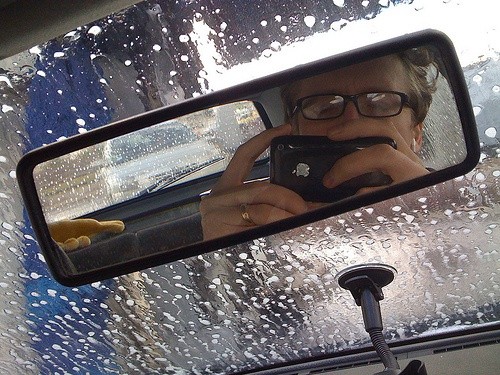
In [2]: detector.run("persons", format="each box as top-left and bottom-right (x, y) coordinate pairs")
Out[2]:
(199, 47), (439, 242)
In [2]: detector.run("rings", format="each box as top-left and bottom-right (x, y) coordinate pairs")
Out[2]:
(240, 204), (256, 227)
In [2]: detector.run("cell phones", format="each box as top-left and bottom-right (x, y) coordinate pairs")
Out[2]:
(268, 134), (396, 205)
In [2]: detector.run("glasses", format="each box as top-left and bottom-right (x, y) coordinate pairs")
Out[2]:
(291, 91), (418, 122)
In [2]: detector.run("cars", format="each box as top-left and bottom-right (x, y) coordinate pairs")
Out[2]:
(101, 121), (225, 207)
